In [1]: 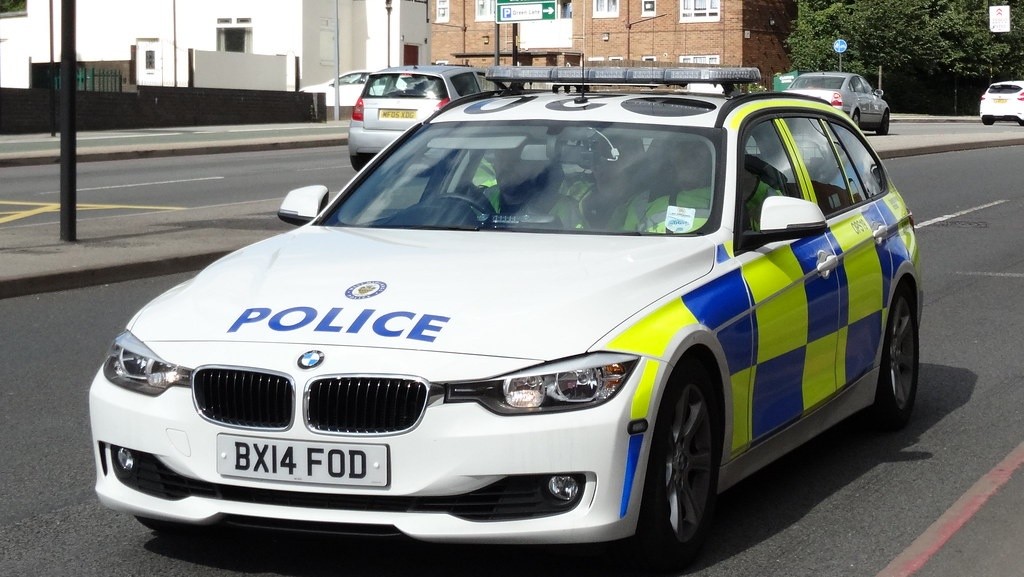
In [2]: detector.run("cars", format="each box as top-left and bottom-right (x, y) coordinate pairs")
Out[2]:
(980, 81), (1024, 126)
(88, 65), (920, 577)
(348, 65), (508, 172)
(782, 73), (890, 135)
(298, 70), (414, 107)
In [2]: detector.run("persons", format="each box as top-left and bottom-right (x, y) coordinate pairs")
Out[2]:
(471, 135), (784, 232)
